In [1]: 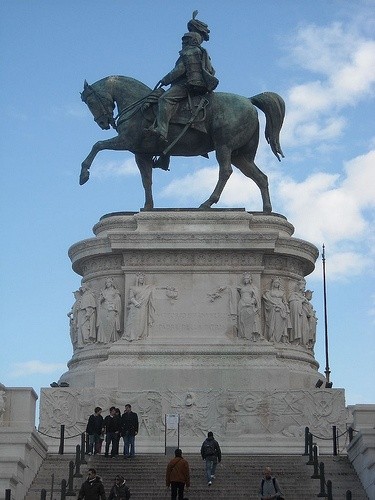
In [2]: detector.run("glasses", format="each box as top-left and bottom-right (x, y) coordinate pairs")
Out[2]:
(114, 479), (119, 481)
(88, 473), (93, 475)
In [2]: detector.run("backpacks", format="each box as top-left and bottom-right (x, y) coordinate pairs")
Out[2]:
(204, 440), (215, 456)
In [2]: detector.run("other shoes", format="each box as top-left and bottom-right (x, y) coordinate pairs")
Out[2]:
(94, 453), (101, 456)
(88, 452), (92, 456)
(105, 452), (108, 457)
(211, 474), (216, 479)
(111, 453), (116, 457)
(130, 452), (135, 455)
(208, 480), (212, 486)
(125, 454), (130, 457)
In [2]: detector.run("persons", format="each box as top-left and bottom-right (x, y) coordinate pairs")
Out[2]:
(258, 468), (284, 500)
(121, 404), (139, 456)
(200, 432), (222, 486)
(228, 272), (319, 351)
(166, 449), (190, 500)
(66, 274), (152, 352)
(77, 468), (107, 500)
(146, 9), (219, 171)
(107, 475), (131, 500)
(87, 406), (121, 457)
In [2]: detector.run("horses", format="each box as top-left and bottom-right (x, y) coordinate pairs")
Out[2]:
(79, 75), (286, 212)
